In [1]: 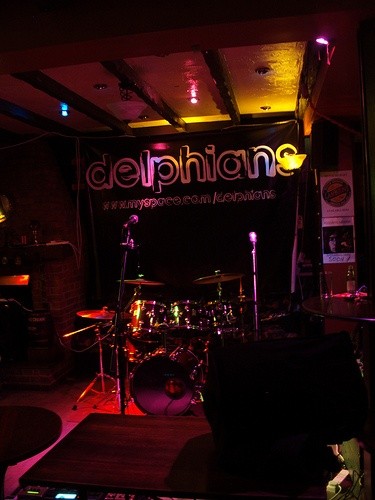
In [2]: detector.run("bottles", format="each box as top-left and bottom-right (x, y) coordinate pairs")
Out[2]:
(346, 264), (355, 302)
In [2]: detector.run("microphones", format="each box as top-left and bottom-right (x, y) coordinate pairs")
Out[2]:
(123, 215), (139, 228)
(249, 232), (257, 243)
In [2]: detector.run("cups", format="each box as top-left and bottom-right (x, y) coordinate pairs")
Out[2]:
(320, 271), (332, 301)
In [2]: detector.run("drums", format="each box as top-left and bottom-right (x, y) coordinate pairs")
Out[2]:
(123, 298), (240, 416)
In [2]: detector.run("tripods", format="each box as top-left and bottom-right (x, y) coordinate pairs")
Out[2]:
(63, 321), (116, 411)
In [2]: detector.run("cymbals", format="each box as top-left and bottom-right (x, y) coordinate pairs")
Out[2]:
(117, 278), (164, 288)
(190, 272), (243, 285)
(76, 308), (117, 320)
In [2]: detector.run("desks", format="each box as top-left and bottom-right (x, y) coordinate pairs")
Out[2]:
(302, 293), (375, 500)
